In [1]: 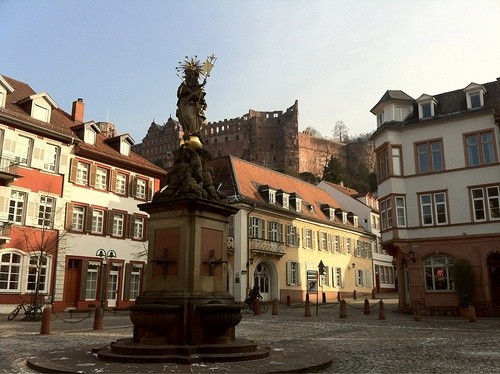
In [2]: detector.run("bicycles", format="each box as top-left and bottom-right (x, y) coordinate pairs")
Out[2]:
(6, 296), (42, 321)
(243, 297), (268, 315)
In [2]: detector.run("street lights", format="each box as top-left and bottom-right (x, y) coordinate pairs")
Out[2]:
(95, 247), (116, 317)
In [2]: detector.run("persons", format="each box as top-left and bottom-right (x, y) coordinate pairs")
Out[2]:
(249, 285), (263, 312)
(175, 69), (207, 141)
